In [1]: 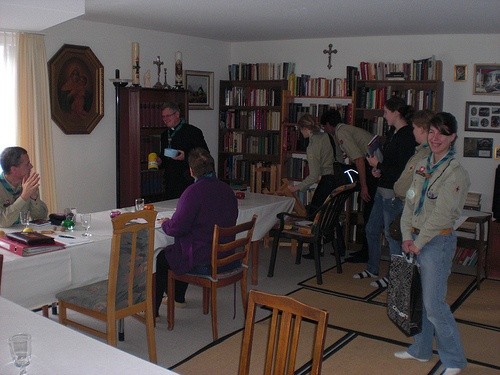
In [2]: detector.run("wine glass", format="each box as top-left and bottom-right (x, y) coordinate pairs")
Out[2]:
(9, 333), (32, 375)
(80, 214), (92, 236)
(19, 211), (31, 228)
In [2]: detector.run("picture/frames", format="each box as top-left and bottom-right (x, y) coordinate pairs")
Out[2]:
(494, 146), (500, 160)
(464, 101), (500, 133)
(464, 137), (493, 159)
(472, 62), (500, 96)
(453, 64), (468, 82)
(46, 44), (105, 135)
(183, 70), (214, 110)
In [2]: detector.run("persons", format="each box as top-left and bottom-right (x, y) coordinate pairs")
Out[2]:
(145, 102), (212, 201)
(0, 147), (48, 294)
(394, 112), (473, 375)
(394, 110), (435, 203)
(286, 114), (347, 258)
(351, 96), (417, 287)
(61, 68), (90, 121)
(319, 107), (385, 264)
(131, 147), (240, 323)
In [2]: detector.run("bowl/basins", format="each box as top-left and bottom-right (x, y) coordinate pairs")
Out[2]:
(164, 148), (179, 158)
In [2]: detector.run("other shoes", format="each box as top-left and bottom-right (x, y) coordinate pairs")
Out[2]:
(136, 310), (161, 323)
(162, 296), (186, 308)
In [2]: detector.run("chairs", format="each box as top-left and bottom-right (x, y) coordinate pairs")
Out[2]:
(54, 209), (158, 364)
(261, 181), (358, 285)
(237, 288), (329, 375)
(452, 210), (492, 289)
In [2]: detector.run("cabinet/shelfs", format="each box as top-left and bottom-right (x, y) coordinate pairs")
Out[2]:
(280, 89), (352, 251)
(116, 87), (189, 209)
(217, 79), (288, 196)
(353, 80), (446, 247)
(486, 220), (500, 281)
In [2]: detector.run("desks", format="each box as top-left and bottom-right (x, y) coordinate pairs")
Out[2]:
(0, 298), (183, 375)
(167, 213), (258, 341)
(0, 189), (296, 317)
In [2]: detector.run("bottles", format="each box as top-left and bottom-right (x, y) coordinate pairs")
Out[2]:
(144, 72), (150, 87)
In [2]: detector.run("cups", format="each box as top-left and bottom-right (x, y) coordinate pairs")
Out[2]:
(64, 208), (77, 222)
(135, 198), (144, 211)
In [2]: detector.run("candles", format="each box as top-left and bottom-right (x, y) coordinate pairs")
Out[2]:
(175, 48), (183, 85)
(131, 42), (140, 84)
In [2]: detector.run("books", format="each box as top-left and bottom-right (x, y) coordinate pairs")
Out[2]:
(465, 192), (481, 208)
(362, 134), (384, 174)
(454, 246), (478, 267)
(454, 221), (479, 241)
(0, 231), (66, 257)
(224, 55), (444, 240)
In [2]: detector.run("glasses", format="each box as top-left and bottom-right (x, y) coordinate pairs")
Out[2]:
(162, 112), (176, 118)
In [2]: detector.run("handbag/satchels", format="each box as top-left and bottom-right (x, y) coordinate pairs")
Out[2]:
(326, 132), (355, 183)
(386, 249), (423, 337)
